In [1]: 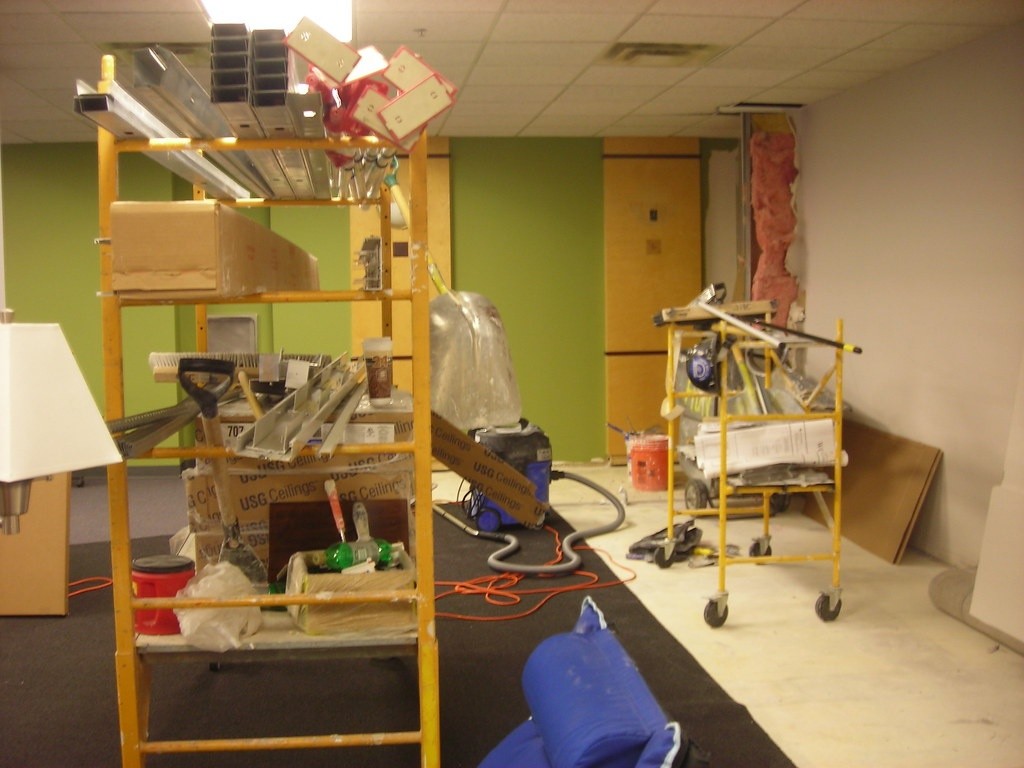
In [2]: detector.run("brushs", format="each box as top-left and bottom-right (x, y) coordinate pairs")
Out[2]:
(147, 351), (333, 420)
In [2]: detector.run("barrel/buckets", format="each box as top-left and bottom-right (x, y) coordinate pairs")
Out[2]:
(627, 435), (668, 491)
(131, 554), (195, 635)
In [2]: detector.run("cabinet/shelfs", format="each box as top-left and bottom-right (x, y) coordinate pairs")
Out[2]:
(97, 55), (441, 768)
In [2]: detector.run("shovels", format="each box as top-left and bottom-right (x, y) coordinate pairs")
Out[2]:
(175, 357), (269, 585)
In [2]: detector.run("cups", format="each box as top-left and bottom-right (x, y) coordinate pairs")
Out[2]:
(365, 337), (394, 406)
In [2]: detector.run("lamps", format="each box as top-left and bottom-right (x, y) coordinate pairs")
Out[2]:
(0, 307), (124, 536)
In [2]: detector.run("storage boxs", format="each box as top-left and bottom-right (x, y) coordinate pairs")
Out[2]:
(284, 542), (415, 636)
(184, 453), (411, 533)
(194, 531), (269, 585)
(109, 199), (320, 301)
(194, 404), (414, 446)
(0, 472), (71, 616)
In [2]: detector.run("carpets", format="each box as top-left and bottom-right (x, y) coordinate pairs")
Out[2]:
(0, 502), (796, 768)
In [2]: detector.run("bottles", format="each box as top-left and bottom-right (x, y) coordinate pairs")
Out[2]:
(312, 539), (392, 573)
